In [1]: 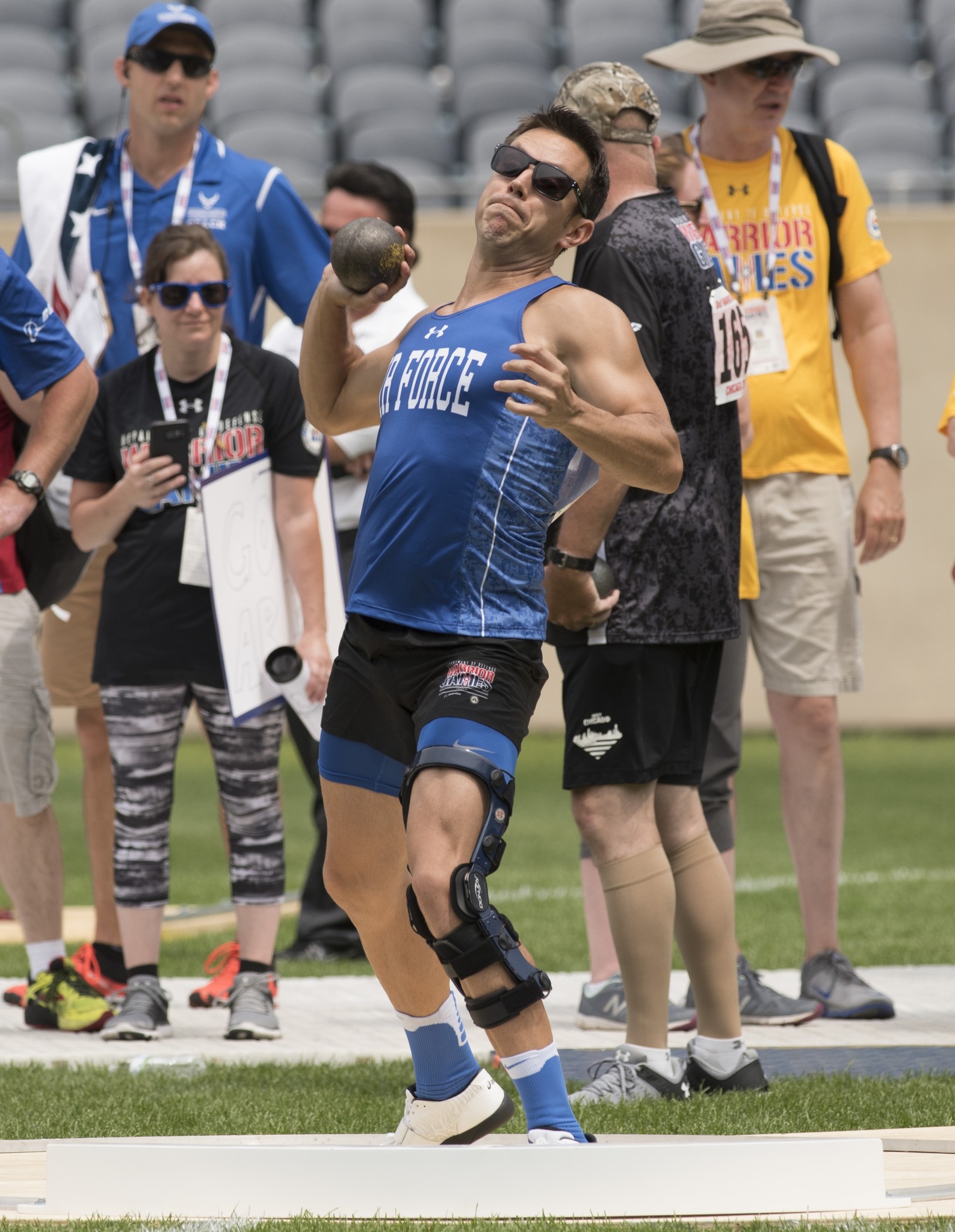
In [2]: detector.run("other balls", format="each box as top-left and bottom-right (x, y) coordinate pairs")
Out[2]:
(329, 216), (405, 294)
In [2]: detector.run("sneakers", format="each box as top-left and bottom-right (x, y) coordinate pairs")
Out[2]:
(99, 975), (176, 1041)
(187, 940), (278, 1008)
(24, 955), (117, 1033)
(568, 1043), (693, 1107)
(679, 1040), (770, 1096)
(574, 968), (692, 1031)
(801, 947), (894, 1020)
(210, 971), (282, 1040)
(684, 955), (824, 1026)
(4, 942), (129, 1009)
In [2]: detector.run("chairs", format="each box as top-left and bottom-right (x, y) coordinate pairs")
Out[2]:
(0, 0), (955, 215)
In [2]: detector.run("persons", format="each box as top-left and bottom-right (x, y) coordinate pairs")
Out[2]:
(53, 224), (326, 1041)
(0, 373), (114, 1030)
(310, 105), (682, 1146)
(0, 247), (98, 540)
(937, 378), (955, 466)
(576, 131), (825, 1032)
(256, 156), (429, 961)
(0, 0), (333, 1016)
(642, 0), (910, 1026)
(541, 62), (770, 1112)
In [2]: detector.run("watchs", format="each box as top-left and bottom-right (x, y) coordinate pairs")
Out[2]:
(6, 468), (44, 504)
(869, 445), (909, 469)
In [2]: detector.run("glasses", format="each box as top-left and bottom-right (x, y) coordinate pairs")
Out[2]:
(744, 56), (805, 82)
(489, 142), (588, 218)
(147, 280), (232, 310)
(127, 47), (211, 79)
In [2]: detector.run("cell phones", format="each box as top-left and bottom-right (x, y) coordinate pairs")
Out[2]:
(148, 419), (191, 488)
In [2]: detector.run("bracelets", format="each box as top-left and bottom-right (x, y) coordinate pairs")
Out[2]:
(544, 545), (598, 574)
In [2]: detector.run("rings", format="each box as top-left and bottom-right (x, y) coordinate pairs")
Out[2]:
(890, 535), (900, 542)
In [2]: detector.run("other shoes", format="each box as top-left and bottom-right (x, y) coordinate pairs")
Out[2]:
(274, 938), (345, 964)
(381, 1068), (515, 1147)
(529, 1127), (599, 1145)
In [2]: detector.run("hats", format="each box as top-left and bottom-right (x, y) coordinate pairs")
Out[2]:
(125, 3), (217, 56)
(552, 61), (662, 146)
(641, 0), (840, 77)
